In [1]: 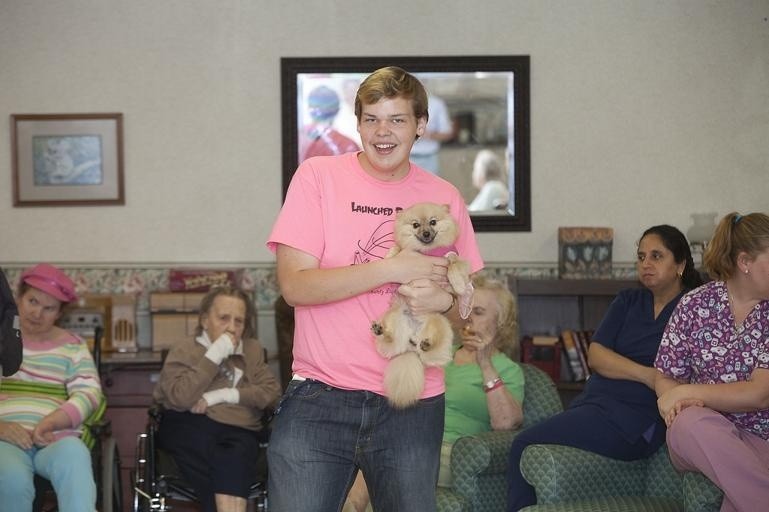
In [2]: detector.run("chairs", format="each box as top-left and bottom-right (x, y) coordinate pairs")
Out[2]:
(425, 363), (563, 512)
(519, 424), (725, 512)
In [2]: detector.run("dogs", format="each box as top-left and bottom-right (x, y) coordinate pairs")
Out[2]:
(370, 203), (474, 412)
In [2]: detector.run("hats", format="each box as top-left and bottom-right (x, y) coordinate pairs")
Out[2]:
(23, 263), (78, 304)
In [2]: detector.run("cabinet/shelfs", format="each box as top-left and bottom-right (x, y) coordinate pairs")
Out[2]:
(82, 349), (162, 512)
(510, 273), (643, 413)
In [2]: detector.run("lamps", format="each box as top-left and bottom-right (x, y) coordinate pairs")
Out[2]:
(686, 211), (721, 269)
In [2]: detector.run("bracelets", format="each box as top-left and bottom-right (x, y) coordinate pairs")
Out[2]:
(484, 376), (505, 393)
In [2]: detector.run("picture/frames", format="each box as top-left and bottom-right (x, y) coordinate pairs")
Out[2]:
(8, 111), (128, 208)
(280, 51), (534, 233)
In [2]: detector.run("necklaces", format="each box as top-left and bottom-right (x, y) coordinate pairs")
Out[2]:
(725, 285), (761, 331)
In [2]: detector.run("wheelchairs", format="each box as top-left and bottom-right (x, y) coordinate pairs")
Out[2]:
(132, 404), (267, 512)
(33, 325), (121, 512)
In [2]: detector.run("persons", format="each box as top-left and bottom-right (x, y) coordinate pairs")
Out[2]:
(297, 85), (362, 160)
(0, 260), (103, 511)
(505, 224), (702, 512)
(339, 277), (525, 512)
(406, 88), (456, 175)
(263, 66), (486, 512)
(154, 284), (286, 511)
(463, 149), (512, 212)
(46, 137), (78, 178)
(654, 208), (768, 512)
